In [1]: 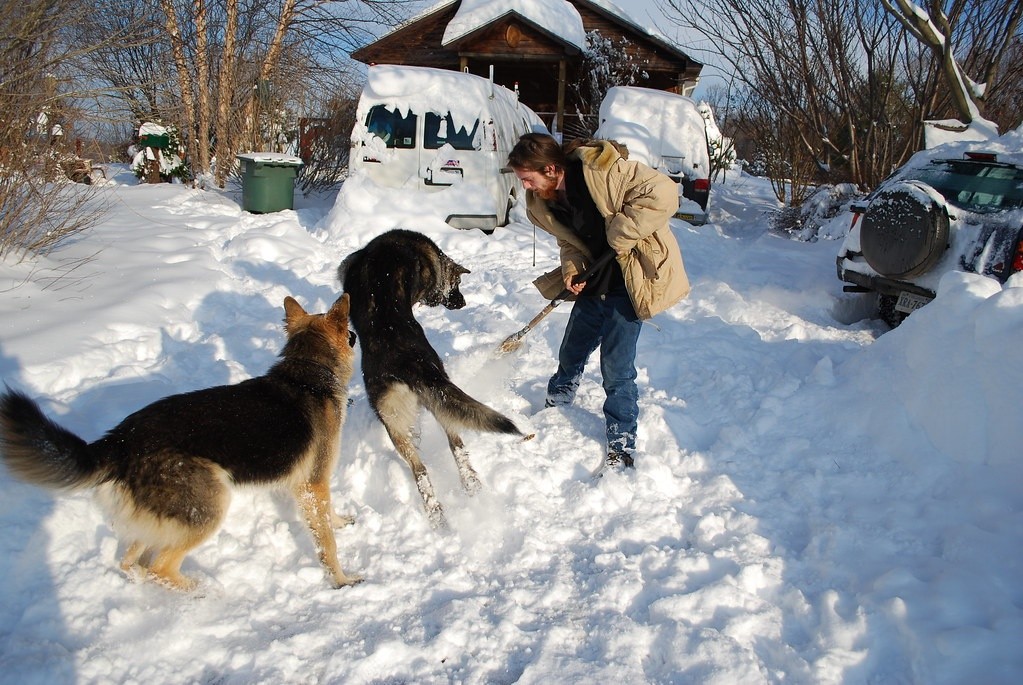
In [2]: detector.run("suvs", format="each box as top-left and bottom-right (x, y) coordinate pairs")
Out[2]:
(593, 86), (711, 227)
(836, 139), (1023, 330)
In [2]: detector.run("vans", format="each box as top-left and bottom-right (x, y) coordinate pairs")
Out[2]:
(348, 67), (547, 233)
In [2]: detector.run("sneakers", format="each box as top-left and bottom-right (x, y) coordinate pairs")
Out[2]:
(596, 452), (633, 478)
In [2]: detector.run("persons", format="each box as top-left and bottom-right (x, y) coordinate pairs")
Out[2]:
(508, 133), (691, 472)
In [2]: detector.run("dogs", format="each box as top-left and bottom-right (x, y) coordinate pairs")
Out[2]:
(0, 294), (366, 589)
(338, 228), (525, 537)
